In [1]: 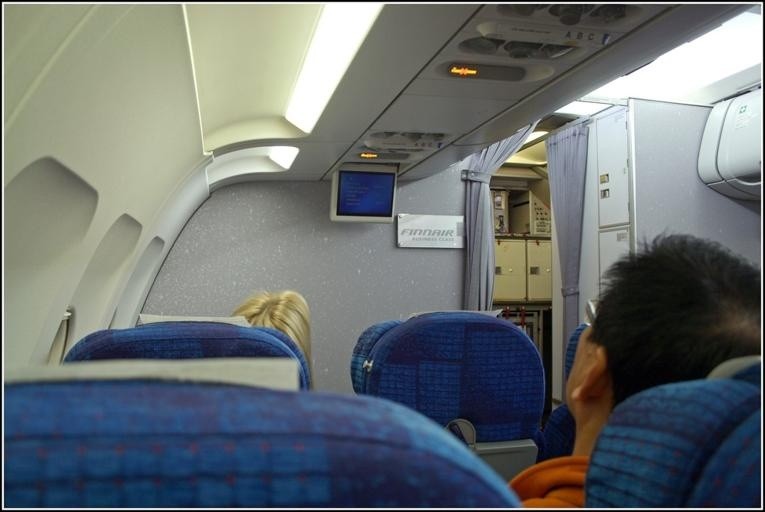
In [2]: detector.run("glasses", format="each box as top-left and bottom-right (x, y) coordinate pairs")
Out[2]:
(583, 299), (600, 327)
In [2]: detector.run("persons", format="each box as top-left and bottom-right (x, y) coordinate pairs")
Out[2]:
(231, 290), (314, 391)
(507, 232), (760, 509)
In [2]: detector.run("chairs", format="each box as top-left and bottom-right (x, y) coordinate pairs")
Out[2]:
(564, 323), (589, 374)
(585, 355), (759, 508)
(65, 323), (311, 397)
(4, 381), (520, 506)
(349, 312), (544, 442)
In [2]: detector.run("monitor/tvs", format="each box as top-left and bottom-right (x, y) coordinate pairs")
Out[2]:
(330, 167), (397, 223)
